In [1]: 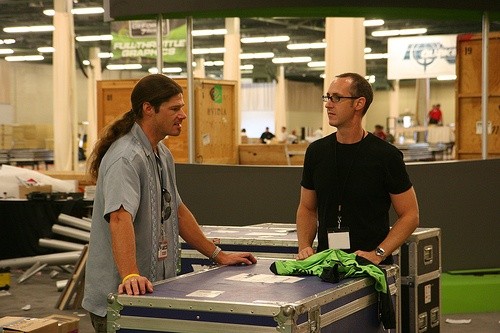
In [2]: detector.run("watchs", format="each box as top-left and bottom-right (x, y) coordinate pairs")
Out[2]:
(374, 247), (385, 261)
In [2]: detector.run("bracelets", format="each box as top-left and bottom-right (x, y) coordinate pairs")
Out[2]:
(122, 272), (140, 282)
(209, 246), (221, 260)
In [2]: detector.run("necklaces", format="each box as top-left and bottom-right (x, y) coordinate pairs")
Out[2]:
(362, 129), (365, 139)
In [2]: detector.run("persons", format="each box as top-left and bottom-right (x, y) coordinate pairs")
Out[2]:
(81, 74), (258, 333)
(260, 126), (323, 145)
(427, 104), (443, 124)
(295, 72), (419, 329)
(372, 124), (397, 144)
(241, 128), (248, 144)
(78, 133), (87, 161)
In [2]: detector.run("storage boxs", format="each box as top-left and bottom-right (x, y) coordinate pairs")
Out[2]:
(18, 185), (52, 199)
(96, 78), (239, 164)
(0, 313), (80, 333)
(107, 222), (441, 333)
(238, 143), (307, 165)
(455, 31), (500, 160)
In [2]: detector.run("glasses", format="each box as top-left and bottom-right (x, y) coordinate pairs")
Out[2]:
(161, 187), (172, 221)
(321, 95), (360, 102)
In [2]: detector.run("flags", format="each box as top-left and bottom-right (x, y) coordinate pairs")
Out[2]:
(110, 18), (188, 63)
(386, 34), (458, 79)
